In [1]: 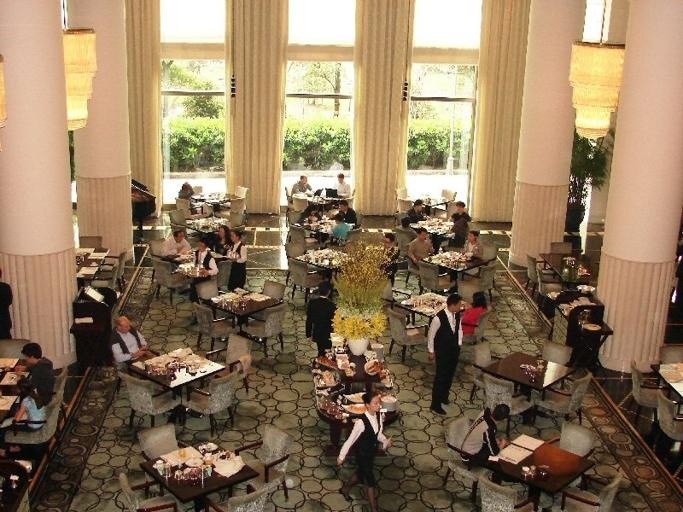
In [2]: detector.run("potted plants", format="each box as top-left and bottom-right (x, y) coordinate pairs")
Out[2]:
(565, 126), (608, 232)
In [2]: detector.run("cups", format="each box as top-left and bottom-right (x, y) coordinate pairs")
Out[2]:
(142, 351), (209, 376)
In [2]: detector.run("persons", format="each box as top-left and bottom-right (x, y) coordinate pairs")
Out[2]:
(304, 280), (338, 357)
(328, 199), (357, 227)
(108, 315), (155, 379)
(333, 173), (353, 202)
(212, 226), (230, 259)
(189, 241), (219, 326)
(160, 230), (191, 269)
(460, 291), (487, 336)
(296, 202), (328, 249)
(289, 175), (316, 202)
(336, 392), (392, 511)
(14, 344), (55, 406)
(460, 402), (511, 486)
(427, 294), (464, 414)
(381, 199), (481, 294)
(178, 182), (199, 217)
(328, 212), (352, 245)
(226, 229), (246, 291)
(0, 270), (15, 341)
(0, 378), (45, 458)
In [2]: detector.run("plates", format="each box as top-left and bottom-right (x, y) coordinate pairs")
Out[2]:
(314, 340), (398, 414)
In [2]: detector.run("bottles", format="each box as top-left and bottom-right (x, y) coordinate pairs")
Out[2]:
(154, 443), (219, 489)
(521, 465), (552, 482)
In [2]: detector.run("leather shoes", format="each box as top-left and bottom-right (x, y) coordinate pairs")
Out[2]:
(430, 403), (447, 415)
(442, 397), (450, 405)
(339, 488), (352, 502)
